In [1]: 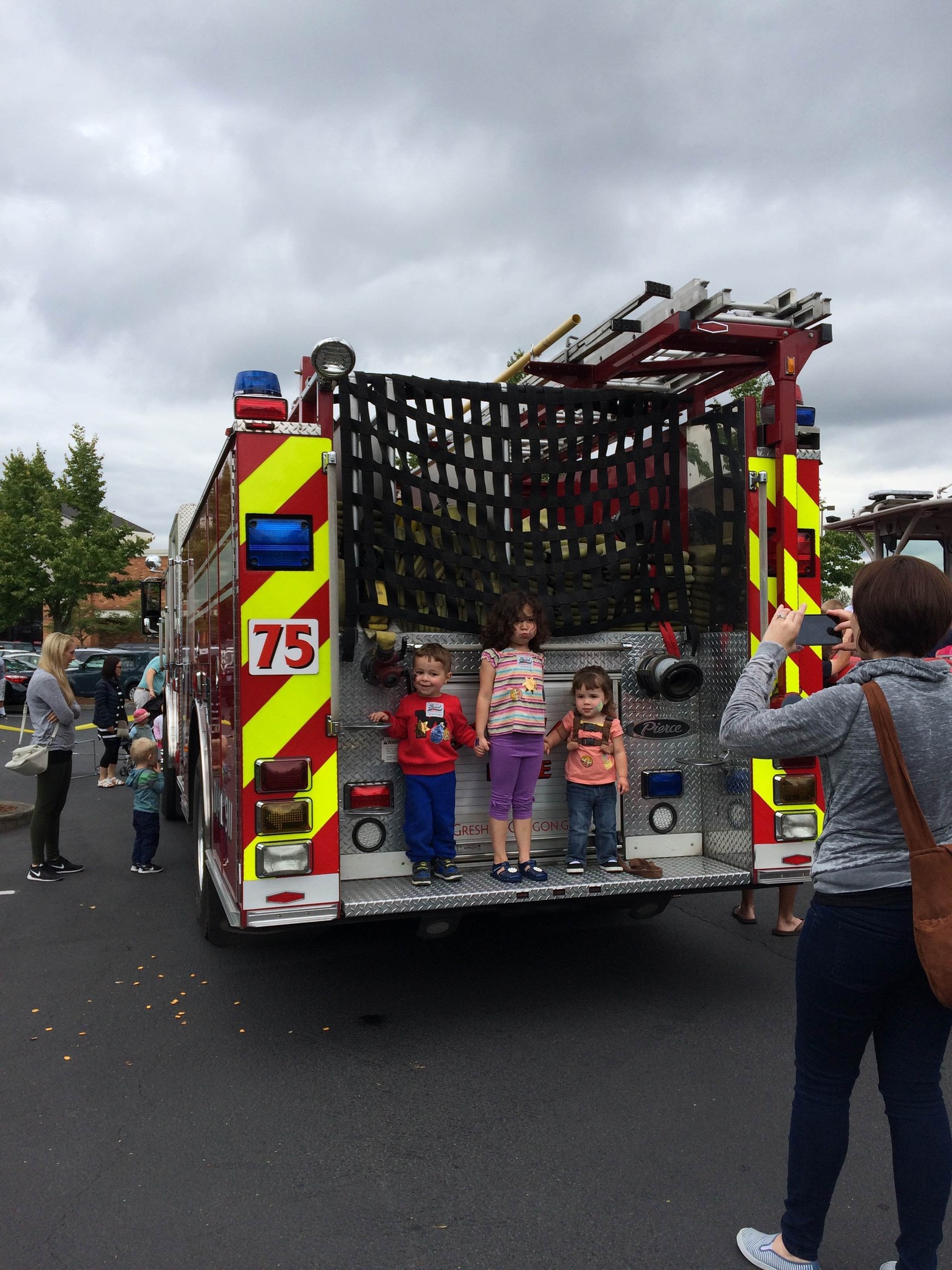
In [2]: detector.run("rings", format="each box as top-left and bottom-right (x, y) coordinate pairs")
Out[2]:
(777, 613), (786, 619)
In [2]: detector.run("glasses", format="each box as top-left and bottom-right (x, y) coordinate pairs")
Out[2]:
(116, 666), (122, 669)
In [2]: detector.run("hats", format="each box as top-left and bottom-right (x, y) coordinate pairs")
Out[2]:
(133, 708), (151, 723)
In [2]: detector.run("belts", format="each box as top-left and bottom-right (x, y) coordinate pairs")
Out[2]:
(137, 687), (150, 692)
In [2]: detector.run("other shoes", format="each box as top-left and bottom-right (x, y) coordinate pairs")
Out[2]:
(599, 860), (623, 872)
(736, 1227), (821, 1270)
(411, 861), (432, 886)
(433, 856), (463, 881)
(566, 861), (584, 873)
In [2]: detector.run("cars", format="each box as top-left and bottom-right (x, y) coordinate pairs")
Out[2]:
(0, 640), (159, 713)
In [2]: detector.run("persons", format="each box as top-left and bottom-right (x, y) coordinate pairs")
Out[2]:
(720, 555), (952, 1269)
(133, 648), (170, 710)
(153, 700), (163, 770)
(368, 642), (490, 886)
(0, 657), (7, 718)
(731, 628), (853, 937)
(26, 633), (84, 882)
(544, 665), (629, 872)
(474, 591), (548, 883)
(126, 708), (156, 743)
(92, 657), (125, 788)
(125, 737), (164, 874)
(821, 598), (844, 615)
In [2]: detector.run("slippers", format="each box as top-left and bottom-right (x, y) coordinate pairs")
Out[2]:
(732, 905), (758, 924)
(774, 918), (806, 936)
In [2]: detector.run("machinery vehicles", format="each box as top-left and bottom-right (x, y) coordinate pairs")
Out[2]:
(822, 484), (952, 680)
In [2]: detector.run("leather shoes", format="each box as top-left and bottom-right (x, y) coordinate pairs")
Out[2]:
(0, 713), (7, 718)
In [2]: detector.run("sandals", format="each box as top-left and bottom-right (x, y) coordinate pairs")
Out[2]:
(490, 861), (523, 883)
(96, 778), (115, 788)
(106, 777), (125, 785)
(517, 859), (548, 881)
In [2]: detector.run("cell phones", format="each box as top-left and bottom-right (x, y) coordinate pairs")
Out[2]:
(796, 614), (843, 646)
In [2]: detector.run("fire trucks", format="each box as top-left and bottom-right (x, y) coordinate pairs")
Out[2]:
(158, 278), (834, 947)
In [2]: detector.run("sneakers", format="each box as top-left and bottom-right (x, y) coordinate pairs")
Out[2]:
(46, 855), (84, 874)
(130, 861), (140, 872)
(27, 862), (64, 882)
(138, 863), (164, 874)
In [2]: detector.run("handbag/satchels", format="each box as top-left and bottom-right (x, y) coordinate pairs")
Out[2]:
(4, 744), (49, 776)
(909, 843), (952, 1009)
(116, 720), (129, 738)
